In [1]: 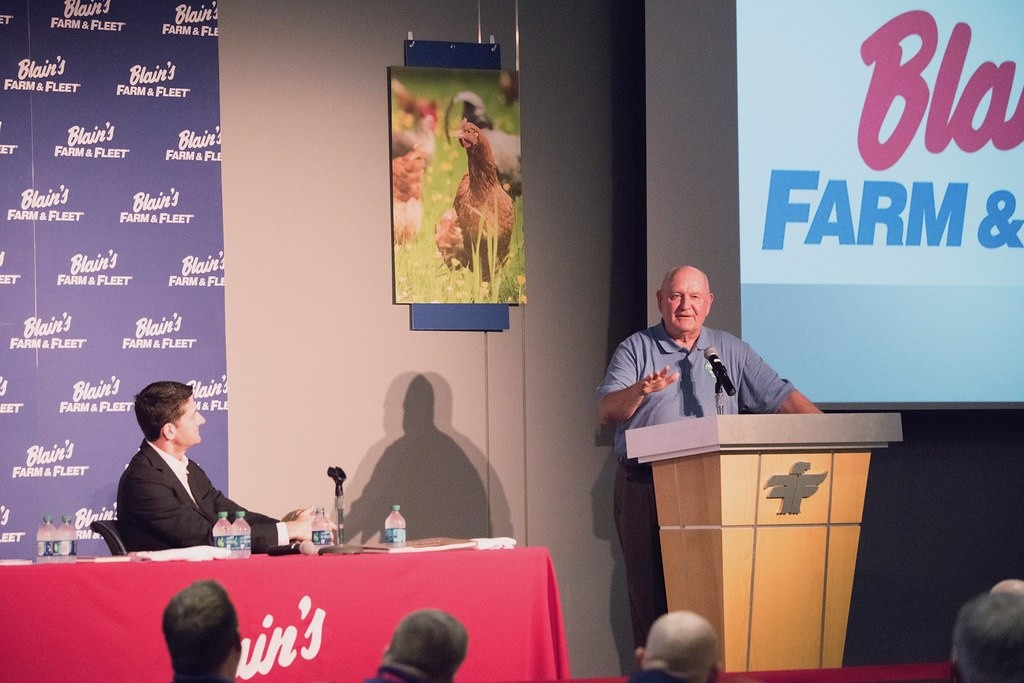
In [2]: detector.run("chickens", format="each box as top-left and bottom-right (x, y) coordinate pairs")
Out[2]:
(389, 70), (521, 283)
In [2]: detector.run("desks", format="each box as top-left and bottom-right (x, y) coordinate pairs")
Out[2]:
(1, 537), (572, 681)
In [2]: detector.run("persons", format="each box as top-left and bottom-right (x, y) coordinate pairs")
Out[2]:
(597, 266), (824, 651)
(362, 610), (468, 683)
(163, 580), (242, 683)
(116, 381), (343, 555)
(951, 579), (1024, 682)
(625, 611), (722, 683)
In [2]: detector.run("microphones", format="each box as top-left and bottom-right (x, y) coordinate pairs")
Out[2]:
(704, 347), (736, 396)
(267, 541), (315, 556)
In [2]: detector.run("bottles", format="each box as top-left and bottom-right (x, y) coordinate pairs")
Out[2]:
(212, 511), (234, 559)
(36, 515), (59, 564)
(384, 506), (407, 543)
(55, 515), (77, 563)
(312, 507), (332, 549)
(229, 511), (251, 559)
